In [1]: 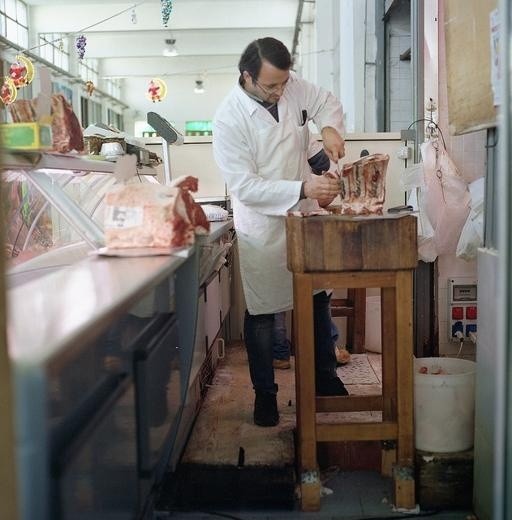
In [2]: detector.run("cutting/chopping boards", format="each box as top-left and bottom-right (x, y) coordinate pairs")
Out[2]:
(284, 207), (418, 272)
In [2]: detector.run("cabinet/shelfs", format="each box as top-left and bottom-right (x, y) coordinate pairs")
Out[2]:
(3, 146), (235, 518)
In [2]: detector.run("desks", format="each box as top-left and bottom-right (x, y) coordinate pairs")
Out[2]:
(291, 271), (418, 513)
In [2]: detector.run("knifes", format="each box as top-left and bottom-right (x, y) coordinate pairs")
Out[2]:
(351, 204), (414, 221)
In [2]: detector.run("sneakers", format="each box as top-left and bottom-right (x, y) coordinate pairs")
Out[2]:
(253, 390), (280, 427)
(318, 385), (349, 397)
(337, 349), (351, 365)
(272, 359), (290, 370)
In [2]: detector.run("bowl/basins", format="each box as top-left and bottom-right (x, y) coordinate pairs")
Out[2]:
(97, 141), (125, 162)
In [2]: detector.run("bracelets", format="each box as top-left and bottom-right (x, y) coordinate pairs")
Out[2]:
(299, 180), (308, 201)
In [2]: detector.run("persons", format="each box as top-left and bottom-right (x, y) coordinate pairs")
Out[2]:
(211, 36), (349, 429)
(269, 123), (353, 372)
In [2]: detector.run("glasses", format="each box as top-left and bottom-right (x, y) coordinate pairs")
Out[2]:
(251, 73), (294, 96)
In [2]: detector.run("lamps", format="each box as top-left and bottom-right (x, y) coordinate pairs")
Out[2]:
(160, 38), (206, 95)
